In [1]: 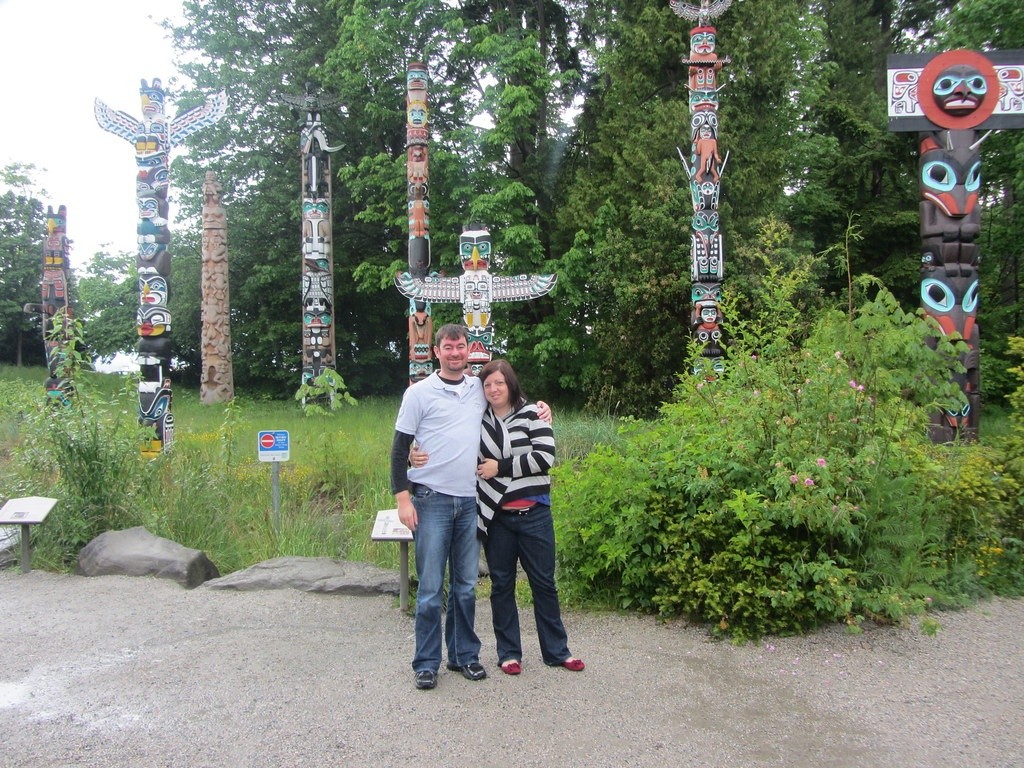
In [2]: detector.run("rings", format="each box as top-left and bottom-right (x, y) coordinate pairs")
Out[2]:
(482, 474), (486, 478)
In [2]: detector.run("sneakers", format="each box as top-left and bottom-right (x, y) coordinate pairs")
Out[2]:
(448, 660), (486, 680)
(416, 669), (436, 688)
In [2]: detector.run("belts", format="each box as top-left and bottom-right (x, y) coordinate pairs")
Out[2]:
(497, 504), (543, 516)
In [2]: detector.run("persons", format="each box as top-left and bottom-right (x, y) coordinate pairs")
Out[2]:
(408, 360), (585, 674)
(391, 323), (553, 690)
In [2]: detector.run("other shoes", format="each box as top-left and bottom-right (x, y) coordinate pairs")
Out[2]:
(502, 662), (522, 674)
(563, 659), (585, 671)
